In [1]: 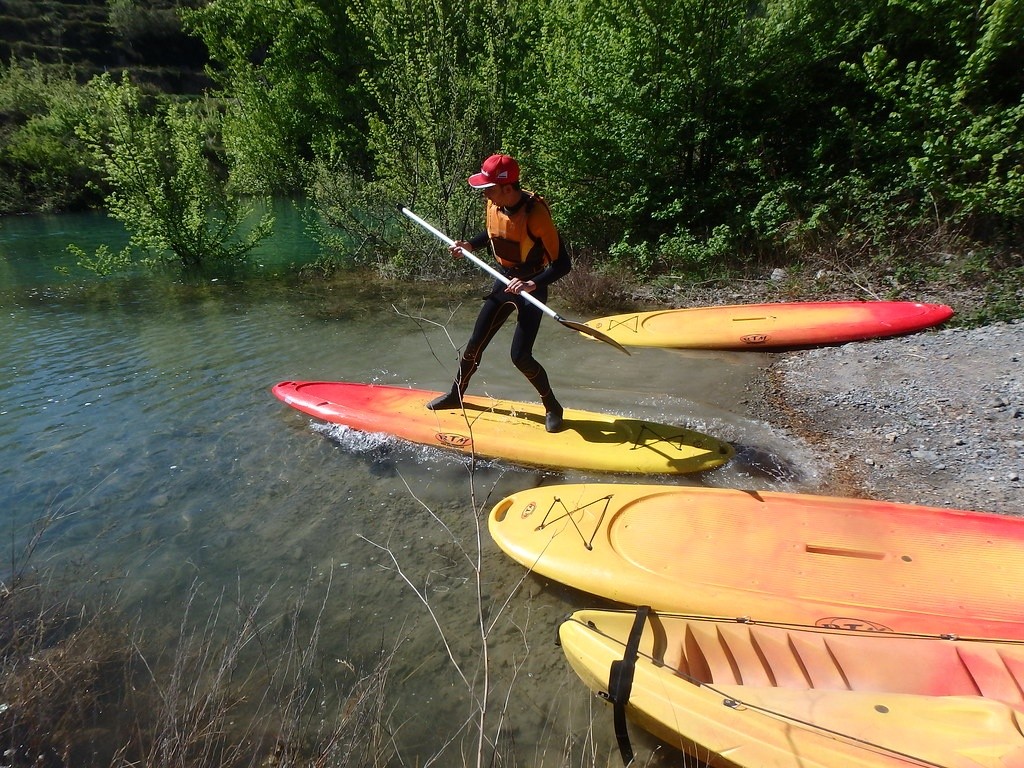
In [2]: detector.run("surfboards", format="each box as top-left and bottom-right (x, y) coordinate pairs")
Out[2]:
(579, 299), (955, 350)
(269, 379), (733, 473)
(489, 480), (1024, 646)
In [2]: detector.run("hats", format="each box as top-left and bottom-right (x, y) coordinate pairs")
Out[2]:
(468, 154), (520, 188)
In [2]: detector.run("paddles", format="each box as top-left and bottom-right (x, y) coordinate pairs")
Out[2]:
(397, 201), (632, 355)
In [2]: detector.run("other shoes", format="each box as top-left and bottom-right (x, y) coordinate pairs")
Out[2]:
(545, 401), (563, 431)
(427, 392), (464, 409)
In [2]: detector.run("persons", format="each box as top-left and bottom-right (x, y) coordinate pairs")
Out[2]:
(426, 155), (571, 433)
